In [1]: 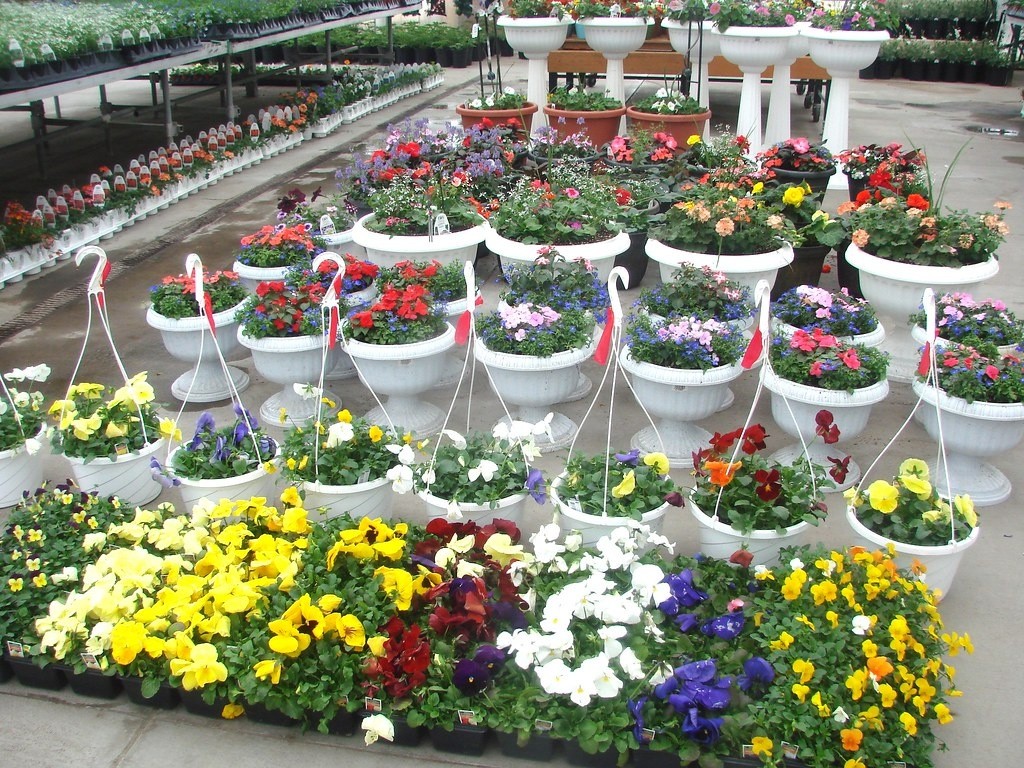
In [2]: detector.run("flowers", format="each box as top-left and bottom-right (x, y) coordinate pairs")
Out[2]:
(0, 0), (1024, 768)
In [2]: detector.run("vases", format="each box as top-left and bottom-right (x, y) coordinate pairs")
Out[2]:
(495, 15), (575, 142)
(624, 107), (712, 157)
(578, 17), (655, 141)
(845, 285), (980, 611)
(845, 243), (999, 382)
(660, 18), (722, 149)
(486, 215), (631, 292)
(146, 297), (248, 403)
(59, 245), (169, 508)
(0, 379), (47, 509)
(645, 237), (795, 368)
(687, 277), (819, 569)
(764, 22), (812, 152)
(543, 104), (627, 154)
(458, 102), (533, 153)
(287, 250), (399, 533)
(712, 23), (800, 157)
(799, 29), (890, 188)
(913, 374), (1024, 508)
(759, 367), (889, 494)
(417, 260), (528, 539)
(617, 345), (745, 469)
(165, 252), (281, 522)
(339, 319), (456, 442)
(475, 336), (599, 455)
(551, 267), (673, 561)
(236, 315), (342, 427)
(231, 259), (296, 298)
(350, 213), (485, 289)
